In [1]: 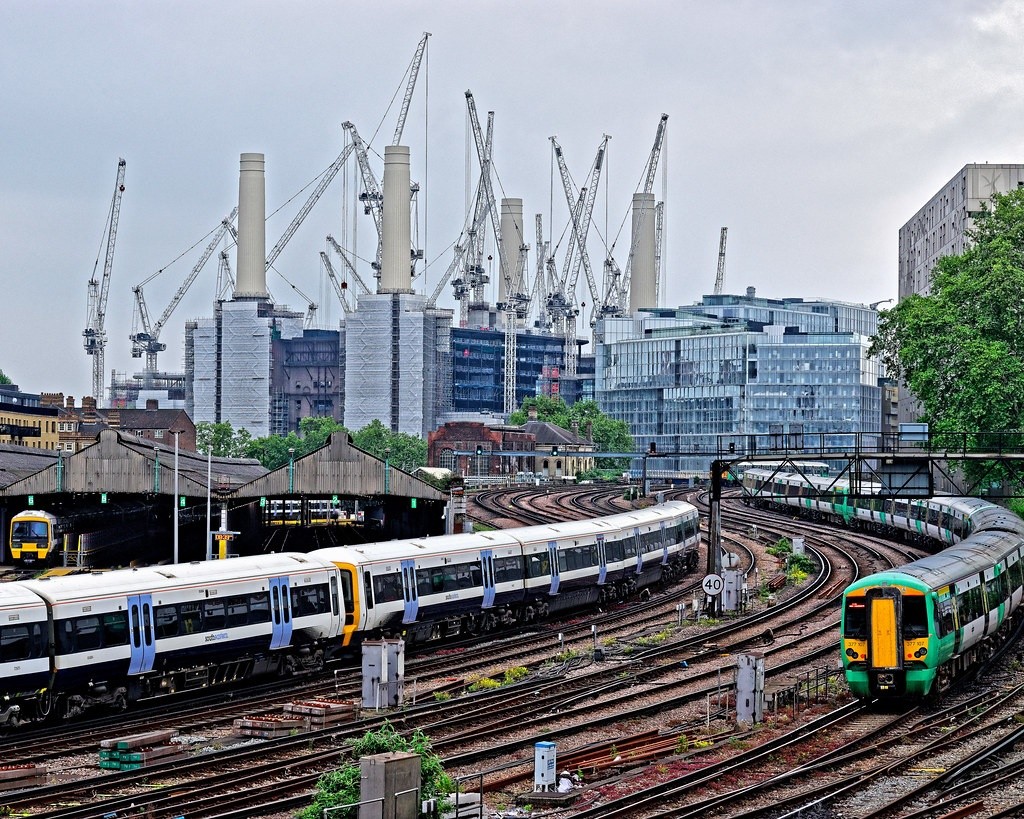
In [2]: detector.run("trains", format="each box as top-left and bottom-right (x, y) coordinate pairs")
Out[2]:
(9, 506), (79, 567)
(719, 465), (829, 486)
(0, 495), (701, 733)
(739, 468), (1024, 702)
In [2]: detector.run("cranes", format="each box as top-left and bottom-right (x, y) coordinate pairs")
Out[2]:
(319, 252), (352, 317)
(338, 115), (423, 296)
(83, 159), (128, 407)
(501, 240), (532, 419)
(210, 143), (354, 306)
(324, 235), (372, 296)
(453, 82), (670, 375)
(711, 228), (729, 298)
(128, 204), (239, 389)
(371, 31), (431, 293)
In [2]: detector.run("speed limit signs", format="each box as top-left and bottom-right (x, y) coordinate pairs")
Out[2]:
(701, 573), (724, 597)
